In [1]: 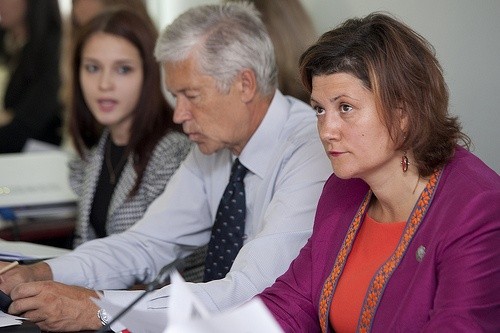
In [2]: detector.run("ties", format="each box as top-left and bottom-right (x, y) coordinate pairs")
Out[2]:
(203, 156), (249, 285)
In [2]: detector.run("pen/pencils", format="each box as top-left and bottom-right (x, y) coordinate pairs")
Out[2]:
(0, 261), (20, 275)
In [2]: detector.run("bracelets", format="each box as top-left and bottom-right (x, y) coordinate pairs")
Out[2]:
(97, 309), (110, 326)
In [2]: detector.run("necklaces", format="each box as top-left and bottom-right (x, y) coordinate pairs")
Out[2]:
(106, 134), (132, 186)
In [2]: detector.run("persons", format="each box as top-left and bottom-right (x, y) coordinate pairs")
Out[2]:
(158, 12), (499, 333)
(69, 9), (212, 291)
(72, 0), (159, 40)
(0, 1), (70, 154)
(0, 1), (335, 333)
(248, 1), (319, 108)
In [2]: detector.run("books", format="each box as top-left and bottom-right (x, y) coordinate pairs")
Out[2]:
(0, 141), (79, 264)
